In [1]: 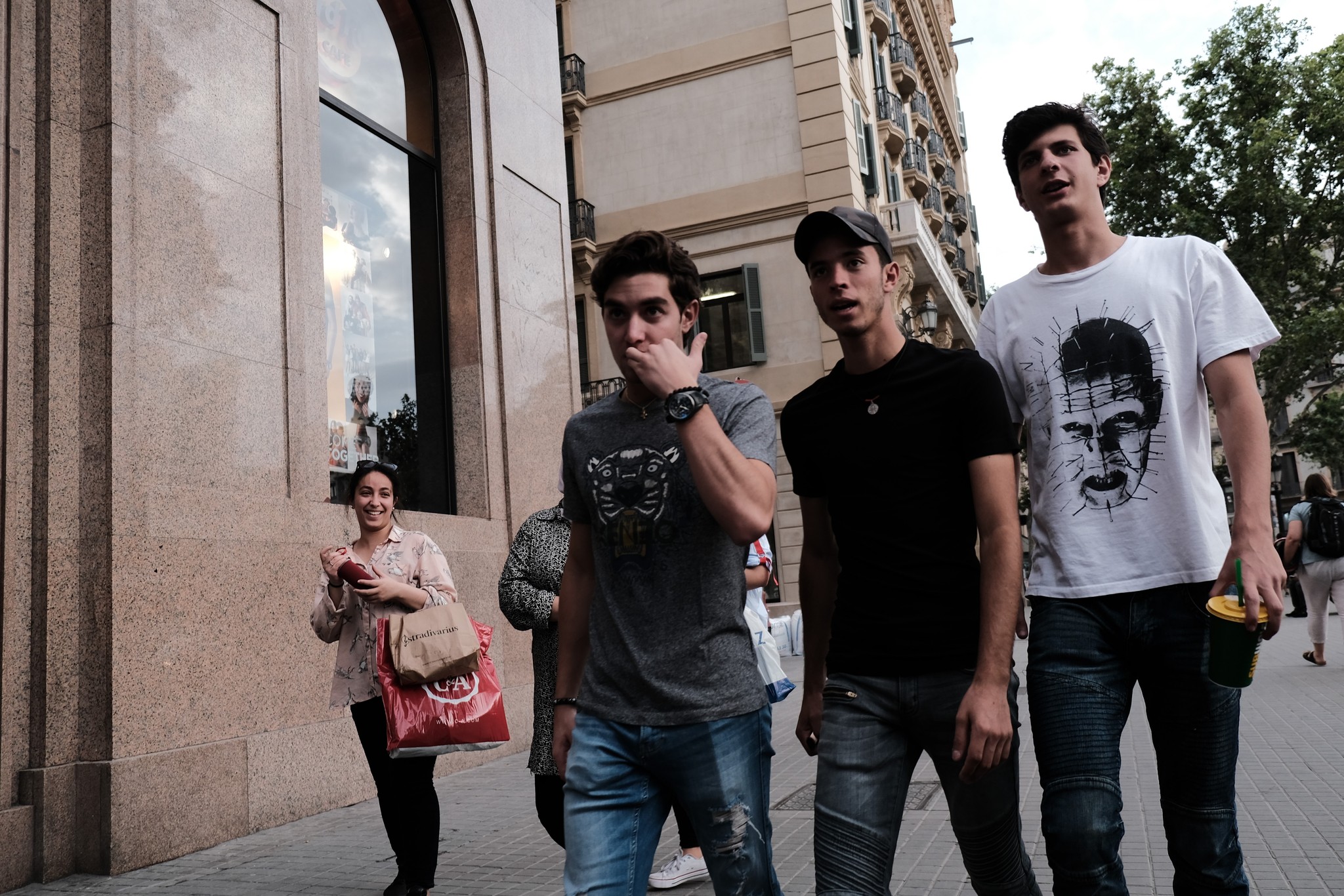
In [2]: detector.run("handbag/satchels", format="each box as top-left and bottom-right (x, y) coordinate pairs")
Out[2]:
(375, 611), (511, 759)
(744, 608), (796, 704)
(389, 587), (480, 689)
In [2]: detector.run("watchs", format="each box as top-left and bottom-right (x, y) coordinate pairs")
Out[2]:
(665, 390), (708, 425)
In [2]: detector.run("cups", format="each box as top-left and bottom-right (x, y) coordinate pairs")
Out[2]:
(1205, 594), (1270, 691)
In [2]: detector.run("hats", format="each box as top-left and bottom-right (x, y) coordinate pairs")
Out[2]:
(794, 206), (894, 261)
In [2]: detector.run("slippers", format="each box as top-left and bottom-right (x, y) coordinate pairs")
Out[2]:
(1303, 650), (1326, 665)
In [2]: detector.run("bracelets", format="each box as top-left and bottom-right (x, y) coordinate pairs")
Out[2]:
(553, 697), (578, 706)
(662, 385), (702, 406)
(330, 579), (344, 588)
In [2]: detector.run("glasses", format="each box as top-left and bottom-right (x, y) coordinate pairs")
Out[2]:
(356, 460), (398, 475)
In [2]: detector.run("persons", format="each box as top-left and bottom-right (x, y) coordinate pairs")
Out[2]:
(553, 231), (785, 896)
(977, 103), (1290, 896)
(1274, 473), (1344, 666)
(313, 459), (458, 896)
(781, 206), (1044, 896)
(496, 455), (579, 850)
(649, 532), (776, 887)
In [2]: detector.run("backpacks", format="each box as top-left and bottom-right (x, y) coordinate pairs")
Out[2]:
(1300, 497), (1343, 559)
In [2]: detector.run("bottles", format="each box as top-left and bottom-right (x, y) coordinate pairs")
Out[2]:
(337, 558), (376, 598)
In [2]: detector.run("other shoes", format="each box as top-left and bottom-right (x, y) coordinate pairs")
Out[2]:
(1285, 611), (1308, 618)
(383, 872), (430, 896)
(1285, 592), (1290, 597)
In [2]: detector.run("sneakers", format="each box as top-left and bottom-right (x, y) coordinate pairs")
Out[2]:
(648, 846), (710, 888)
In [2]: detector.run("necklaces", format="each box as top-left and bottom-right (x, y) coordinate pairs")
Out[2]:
(622, 385), (659, 419)
(837, 345), (909, 413)
